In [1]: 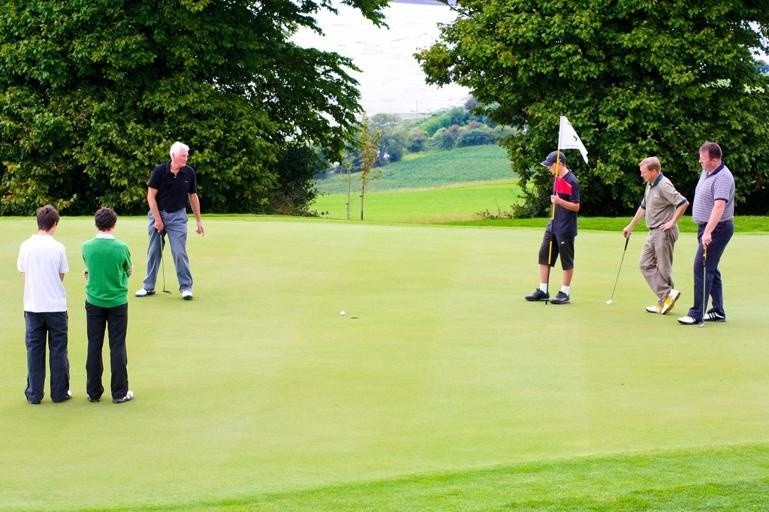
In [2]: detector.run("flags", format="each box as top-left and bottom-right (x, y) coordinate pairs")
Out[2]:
(554, 177), (572, 195)
(558, 115), (588, 165)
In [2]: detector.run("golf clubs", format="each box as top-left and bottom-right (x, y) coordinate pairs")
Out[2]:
(160, 235), (170, 293)
(696, 246), (706, 328)
(606, 233), (631, 304)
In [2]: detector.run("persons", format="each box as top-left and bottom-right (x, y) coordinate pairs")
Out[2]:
(82, 207), (133, 403)
(135, 142), (205, 298)
(622, 156), (689, 315)
(17, 204), (73, 404)
(678, 142), (734, 325)
(525, 150), (581, 304)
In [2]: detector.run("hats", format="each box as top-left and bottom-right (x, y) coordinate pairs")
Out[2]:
(541, 151), (566, 166)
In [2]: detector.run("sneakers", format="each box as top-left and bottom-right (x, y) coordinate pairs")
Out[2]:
(87, 392), (100, 402)
(525, 288), (549, 300)
(113, 390), (133, 403)
(136, 288), (155, 296)
(181, 290), (193, 298)
(63, 390), (73, 400)
(678, 308), (725, 324)
(551, 291), (569, 304)
(647, 289), (680, 314)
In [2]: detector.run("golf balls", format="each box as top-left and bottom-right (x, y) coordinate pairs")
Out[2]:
(340, 311), (345, 315)
